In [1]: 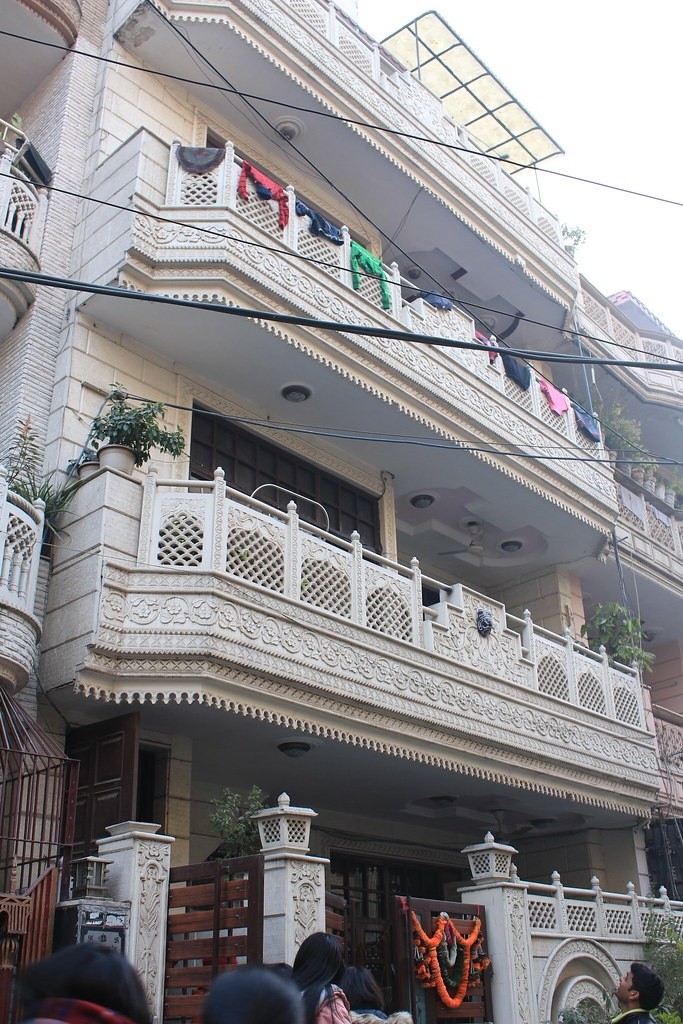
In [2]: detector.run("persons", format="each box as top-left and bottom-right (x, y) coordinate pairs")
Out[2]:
(608, 962), (665, 1024)
(292, 931), (355, 1024)
(199, 962), (307, 1024)
(16, 941), (154, 1024)
(331, 964), (416, 1024)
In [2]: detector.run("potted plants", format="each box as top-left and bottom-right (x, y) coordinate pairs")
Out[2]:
(87, 381), (186, 476)
(583, 386), (683, 511)
(561, 222), (587, 259)
(67, 448), (100, 481)
(189, 784), (272, 1024)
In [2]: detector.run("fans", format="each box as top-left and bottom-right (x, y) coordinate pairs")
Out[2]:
(456, 809), (533, 854)
(437, 521), (515, 563)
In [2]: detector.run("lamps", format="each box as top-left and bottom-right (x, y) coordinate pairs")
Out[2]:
(531, 818), (555, 830)
(641, 631), (657, 642)
(430, 795), (458, 808)
(281, 385), (310, 402)
(476, 317), (495, 335)
(410, 494), (434, 509)
(406, 267), (423, 279)
(501, 541), (523, 553)
(278, 742), (311, 759)
(279, 126), (296, 141)
